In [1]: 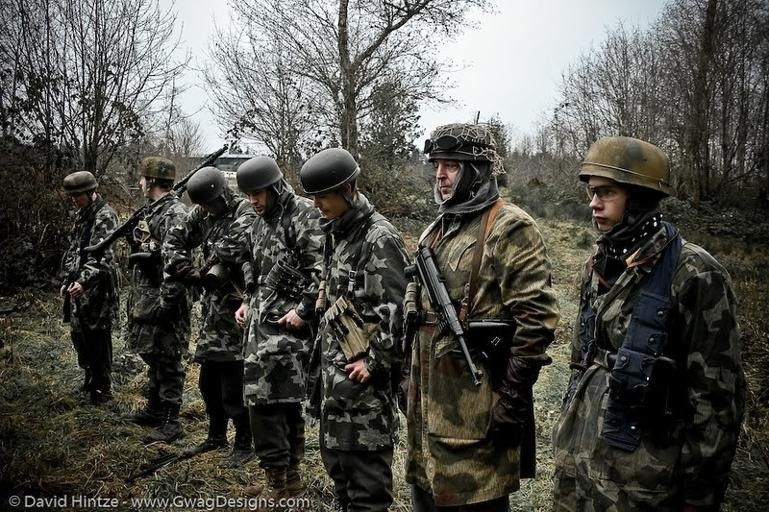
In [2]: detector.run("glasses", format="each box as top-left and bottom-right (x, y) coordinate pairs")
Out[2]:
(423, 134), (459, 154)
(586, 183), (621, 202)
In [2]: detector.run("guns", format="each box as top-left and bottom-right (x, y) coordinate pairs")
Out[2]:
(82, 144), (230, 260)
(404, 248), (484, 388)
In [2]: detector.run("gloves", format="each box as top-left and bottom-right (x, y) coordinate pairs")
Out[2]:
(486, 354), (534, 457)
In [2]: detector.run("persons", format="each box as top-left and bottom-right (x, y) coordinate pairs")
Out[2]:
(62, 170), (122, 406)
(400, 123), (561, 511)
(125, 156), (187, 443)
(161, 165), (255, 470)
(236, 156), (324, 511)
(300, 148), (410, 511)
(551, 136), (747, 511)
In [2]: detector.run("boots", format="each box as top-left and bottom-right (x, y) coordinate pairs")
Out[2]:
(80, 376), (305, 512)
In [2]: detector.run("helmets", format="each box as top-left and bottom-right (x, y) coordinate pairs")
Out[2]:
(63, 171), (99, 193)
(138, 156), (176, 179)
(237, 157), (283, 193)
(187, 166), (226, 204)
(300, 148), (361, 195)
(578, 136), (672, 198)
(427, 123), (497, 162)
